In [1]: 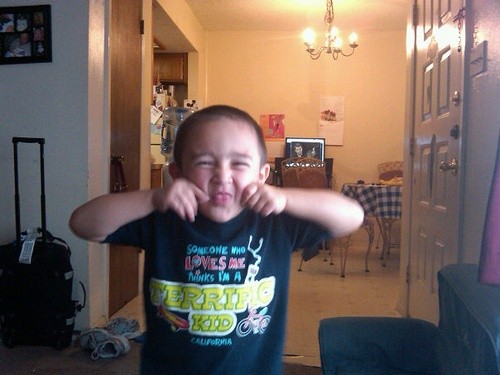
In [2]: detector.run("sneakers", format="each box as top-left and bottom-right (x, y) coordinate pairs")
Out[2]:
(103, 316), (142, 339)
(79, 327), (129, 360)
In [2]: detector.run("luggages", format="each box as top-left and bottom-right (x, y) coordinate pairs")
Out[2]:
(0, 137), (85, 349)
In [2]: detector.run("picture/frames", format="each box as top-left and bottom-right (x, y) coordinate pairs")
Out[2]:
(0, 4), (54, 65)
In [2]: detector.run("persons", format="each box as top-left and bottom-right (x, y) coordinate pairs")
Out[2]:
(68, 106), (364, 375)
(293, 144), (304, 158)
(0, 12), (45, 57)
(311, 147), (320, 159)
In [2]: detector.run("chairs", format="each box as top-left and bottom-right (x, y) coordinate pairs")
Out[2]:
(281, 158), (404, 272)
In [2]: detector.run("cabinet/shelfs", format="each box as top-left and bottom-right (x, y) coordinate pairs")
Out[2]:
(153, 51), (188, 86)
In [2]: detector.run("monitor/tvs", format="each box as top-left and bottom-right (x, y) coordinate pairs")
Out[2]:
(285, 138), (324, 168)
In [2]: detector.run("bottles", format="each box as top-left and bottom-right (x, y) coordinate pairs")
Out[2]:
(160, 107), (194, 164)
(108, 154), (129, 194)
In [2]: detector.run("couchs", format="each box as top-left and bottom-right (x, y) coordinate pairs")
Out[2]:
(318, 264), (500, 375)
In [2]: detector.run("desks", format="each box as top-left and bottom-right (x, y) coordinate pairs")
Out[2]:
(337, 182), (404, 278)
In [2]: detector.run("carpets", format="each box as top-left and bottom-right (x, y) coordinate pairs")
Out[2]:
(0, 338), (324, 375)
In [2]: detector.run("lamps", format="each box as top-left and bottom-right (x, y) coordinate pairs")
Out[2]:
(301, 0), (359, 61)
